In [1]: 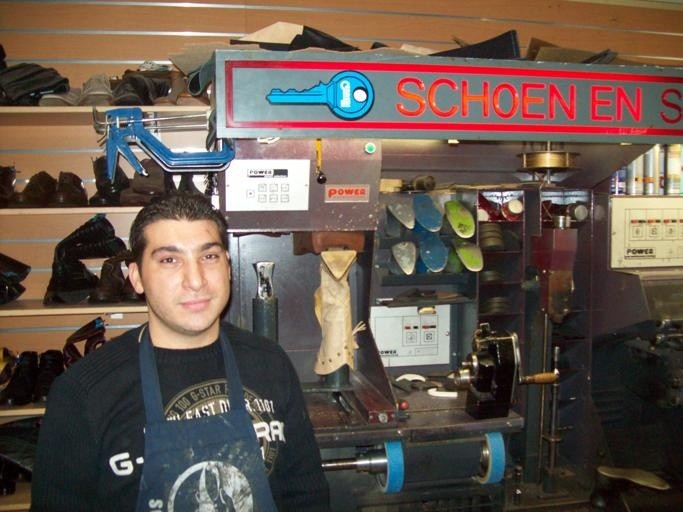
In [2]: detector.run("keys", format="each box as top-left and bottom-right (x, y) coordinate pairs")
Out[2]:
(266, 70), (376, 122)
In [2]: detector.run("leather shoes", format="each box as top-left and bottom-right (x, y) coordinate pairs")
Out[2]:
(130, 75), (170, 104)
(0, 156), (204, 206)
(113, 82), (143, 105)
(0, 351), (37, 408)
(37, 350), (62, 403)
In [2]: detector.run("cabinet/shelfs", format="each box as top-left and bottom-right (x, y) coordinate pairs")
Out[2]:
(0, 105), (214, 512)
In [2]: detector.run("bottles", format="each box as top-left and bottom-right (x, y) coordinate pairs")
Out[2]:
(611, 144), (682, 195)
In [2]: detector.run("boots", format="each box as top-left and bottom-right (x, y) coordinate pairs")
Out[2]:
(0, 62), (69, 106)
(314, 248), (367, 376)
(109, 69), (210, 105)
(0, 214), (138, 304)
(61, 317), (104, 372)
(84, 335), (106, 355)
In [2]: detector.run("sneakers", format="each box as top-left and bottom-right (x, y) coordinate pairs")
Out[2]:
(79, 76), (114, 106)
(38, 87), (81, 106)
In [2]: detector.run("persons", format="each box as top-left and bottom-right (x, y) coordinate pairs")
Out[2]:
(29, 189), (330, 511)
